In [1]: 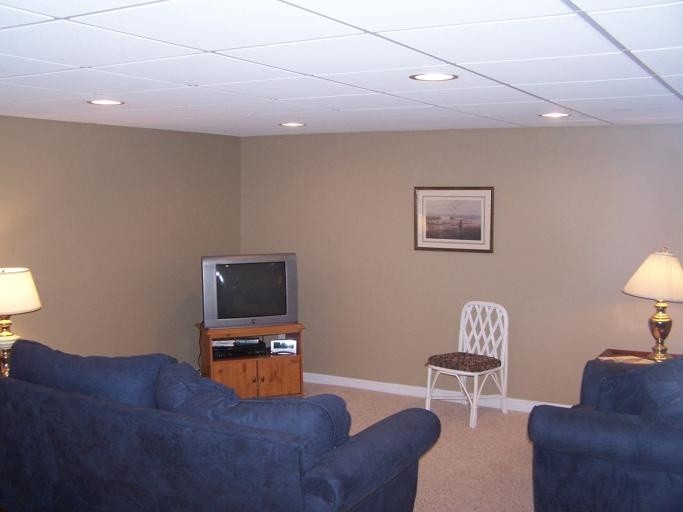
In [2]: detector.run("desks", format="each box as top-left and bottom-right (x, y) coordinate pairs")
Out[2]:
(595, 347), (668, 371)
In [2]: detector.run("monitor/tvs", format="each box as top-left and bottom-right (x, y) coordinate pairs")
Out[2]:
(201, 253), (298, 330)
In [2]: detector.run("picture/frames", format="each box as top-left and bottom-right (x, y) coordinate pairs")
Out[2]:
(412, 184), (496, 255)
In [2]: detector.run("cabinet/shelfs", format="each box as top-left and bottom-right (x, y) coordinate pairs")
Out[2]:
(193, 320), (306, 398)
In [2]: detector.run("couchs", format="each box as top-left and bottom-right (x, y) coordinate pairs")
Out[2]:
(0, 340), (439, 511)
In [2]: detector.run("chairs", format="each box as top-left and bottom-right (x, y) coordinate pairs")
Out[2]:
(524, 357), (681, 512)
(422, 299), (511, 430)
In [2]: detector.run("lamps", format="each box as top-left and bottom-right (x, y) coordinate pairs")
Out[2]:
(2, 265), (44, 339)
(620, 244), (683, 363)
